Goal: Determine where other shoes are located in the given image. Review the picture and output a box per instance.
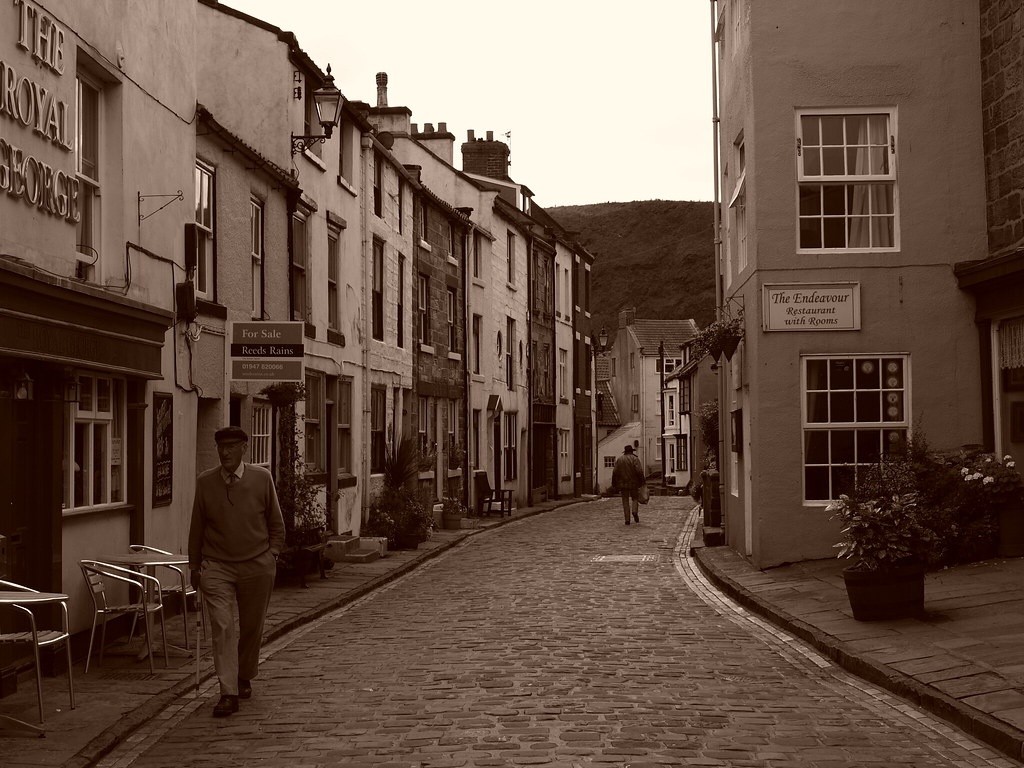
[632,512,639,523]
[625,521,630,525]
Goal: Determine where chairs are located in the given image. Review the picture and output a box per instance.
[78,559,169,675]
[476,471,515,518]
[126,544,207,651]
[0,580,75,723]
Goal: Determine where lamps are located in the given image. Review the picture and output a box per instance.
[590,326,608,356]
[291,63,345,158]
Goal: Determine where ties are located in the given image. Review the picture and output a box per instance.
[229,473,236,482]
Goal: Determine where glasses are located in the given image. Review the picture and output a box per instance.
[225,483,234,506]
[214,442,246,450]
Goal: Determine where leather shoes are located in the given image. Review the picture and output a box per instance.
[238,677,252,698]
[213,695,239,715]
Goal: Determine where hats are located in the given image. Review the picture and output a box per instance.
[622,445,636,453]
[215,426,248,443]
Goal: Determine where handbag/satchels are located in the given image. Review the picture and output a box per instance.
[638,483,650,504]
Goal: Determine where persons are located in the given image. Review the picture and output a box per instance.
[612,445,647,525]
[188,427,285,719]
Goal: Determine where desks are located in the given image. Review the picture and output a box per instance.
[95,556,193,661]
[0,592,70,738]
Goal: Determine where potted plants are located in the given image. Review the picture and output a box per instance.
[701,326,723,362]
[716,321,744,362]
[833,437,962,621]
